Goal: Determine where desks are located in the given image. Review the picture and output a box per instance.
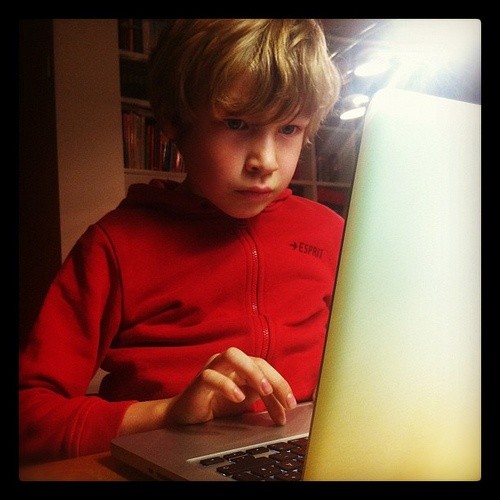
[19,450,154,481]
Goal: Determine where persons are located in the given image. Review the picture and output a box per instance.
[18,18,345,457]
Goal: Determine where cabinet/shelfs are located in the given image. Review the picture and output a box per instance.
[17,18,364,354]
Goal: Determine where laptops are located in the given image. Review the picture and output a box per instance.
[112,84,482,480]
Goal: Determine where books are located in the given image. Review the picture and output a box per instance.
[120,106,188,174]
[119,17,177,52]
[318,135,349,184]
